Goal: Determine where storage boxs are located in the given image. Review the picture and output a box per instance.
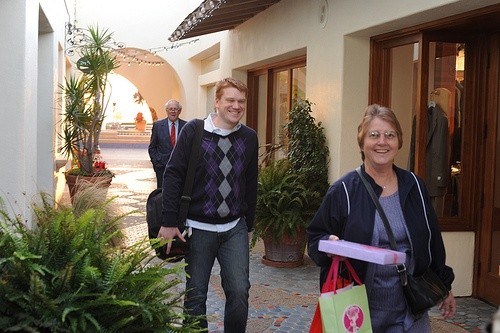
[318,240,407,265]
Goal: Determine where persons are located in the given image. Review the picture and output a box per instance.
[306,104,456,333]
[157,77,259,333]
[148,100,188,188]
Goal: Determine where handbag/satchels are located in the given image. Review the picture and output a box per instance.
[307,255,374,333]
[144,187,192,264]
[399,267,450,315]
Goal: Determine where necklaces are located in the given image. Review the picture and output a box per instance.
[383,186,387,189]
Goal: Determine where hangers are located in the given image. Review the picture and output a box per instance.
[410,91,449,120]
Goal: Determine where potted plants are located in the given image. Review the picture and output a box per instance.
[52,22,121,214]
[249,98,329,268]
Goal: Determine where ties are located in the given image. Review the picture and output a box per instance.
[170,122,176,147]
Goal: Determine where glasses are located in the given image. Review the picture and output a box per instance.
[367,129,398,139]
[169,108,179,113]
